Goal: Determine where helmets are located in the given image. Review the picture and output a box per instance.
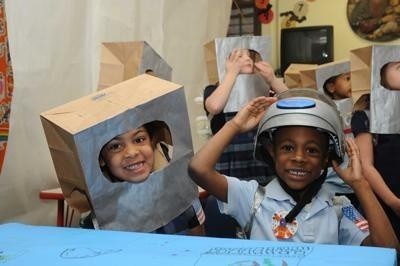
[253,88,346,167]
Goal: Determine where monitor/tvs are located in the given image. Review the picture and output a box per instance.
[281,25,334,78]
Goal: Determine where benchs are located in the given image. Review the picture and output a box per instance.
[39,186,66,227]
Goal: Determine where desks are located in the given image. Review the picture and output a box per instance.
[0,223,398,266]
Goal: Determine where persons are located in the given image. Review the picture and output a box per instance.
[67,46,400,247]
